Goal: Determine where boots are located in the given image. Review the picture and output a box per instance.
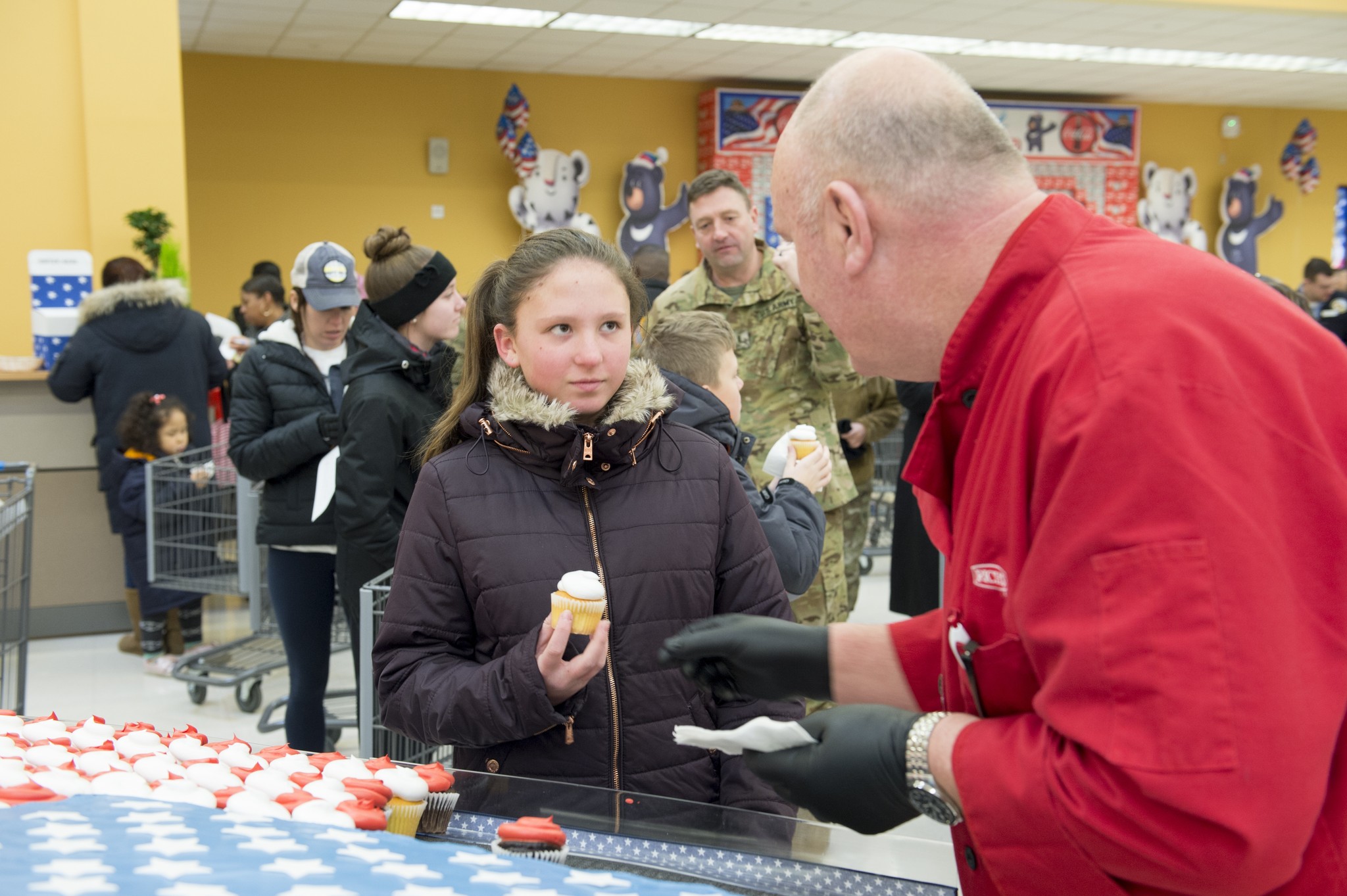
[117,587,186,656]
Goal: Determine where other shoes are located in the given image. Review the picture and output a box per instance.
[142,656,174,675]
[185,645,218,658]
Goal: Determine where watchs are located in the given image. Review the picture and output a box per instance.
[903,709,966,826]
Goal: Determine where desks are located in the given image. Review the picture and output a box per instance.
[0,706,959,895]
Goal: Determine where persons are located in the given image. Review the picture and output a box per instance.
[42,255,231,665]
[1292,257,1334,329]
[819,359,911,632]
[630,167,864,863]
[223,273,298,415]
[638,304,837,611]
[111,388,220,680]
[883,368,947,628]
[1315,249,1347,348]
[371,224,821,879]
[223,258,288,341]
[330,225,476,741]
[222,238,371,763]
[653,42,1347,896]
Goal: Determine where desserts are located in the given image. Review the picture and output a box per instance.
[492,814,569,864]
[0,706,459,838]
[550,570,607,635]
[789,424,819,461]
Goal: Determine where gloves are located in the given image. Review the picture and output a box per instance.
[659,614,832,703]
[718,703,929,837]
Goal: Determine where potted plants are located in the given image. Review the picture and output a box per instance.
[124,205,171,284]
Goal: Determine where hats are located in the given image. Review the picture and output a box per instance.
[292,242,363,310]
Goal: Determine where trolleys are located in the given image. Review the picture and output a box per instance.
[145,441,354,713]
[854,407,912,572]
